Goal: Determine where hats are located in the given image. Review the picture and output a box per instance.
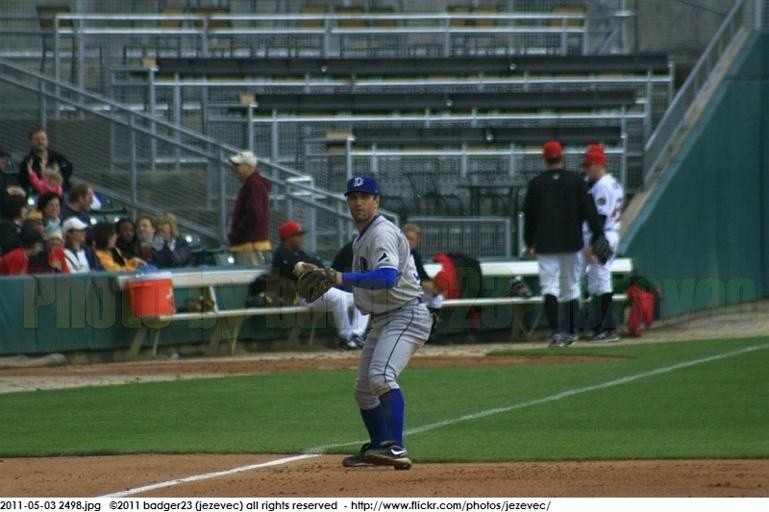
[542,140,563,159]
[279,220,310,239]
[63,215,89,234]
[344,175,379,198]
[229,150,258,168]
[580,144,608,166]
[47,224,63,241]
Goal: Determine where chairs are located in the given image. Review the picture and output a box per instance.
[0,149,239,267]
[353,169,543,218]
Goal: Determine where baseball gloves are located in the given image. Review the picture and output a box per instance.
[295,266,337,304]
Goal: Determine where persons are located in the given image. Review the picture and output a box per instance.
[228,151,272,352]
[402,223,440,343]
[290,173,433,469]
[0,125,192,276]
[522,140,624,349]
[273,220,371,350]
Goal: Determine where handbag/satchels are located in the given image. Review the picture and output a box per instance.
[245,271,303,309]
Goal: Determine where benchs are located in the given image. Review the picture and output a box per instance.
[113,250,636,358]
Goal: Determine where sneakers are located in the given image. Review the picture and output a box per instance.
[547,334,564,348]
[342,442,387,468]
[563,334,578,348]
[337,337,358,352]
[588,330,620,344]
[363,444,413,471]
[352,335,374,350]
